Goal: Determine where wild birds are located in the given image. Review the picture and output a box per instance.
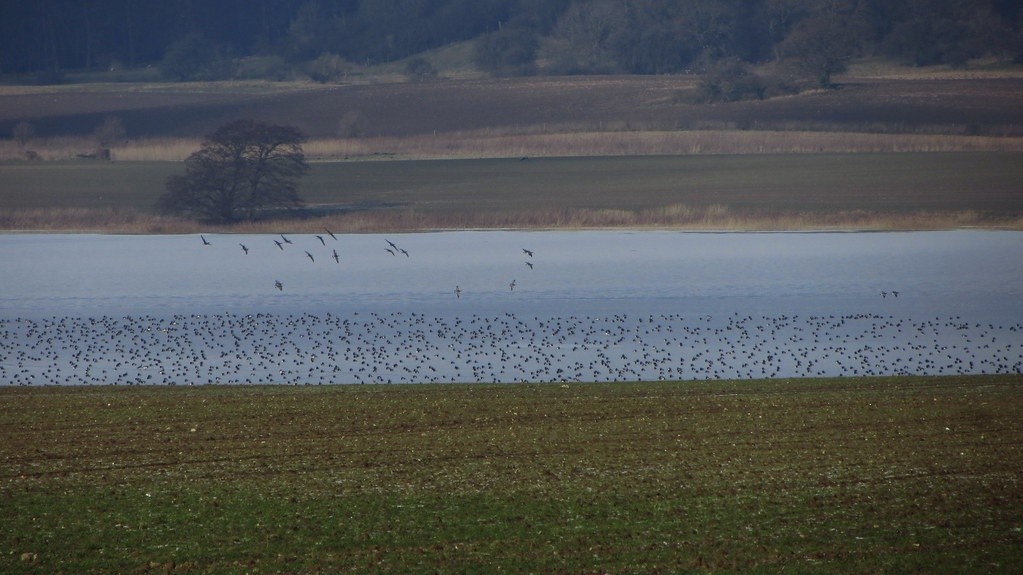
[399,248,409,257]
[892,290,899,297]
[315,235,325,246]
[332,249,341,263]
[240,243,249,255]
[384,248,395,257]
[201,235,214,245]
[304,250,314,263]
[454,285,464,299]
[880,291,887,298]
[509,280,516,291]
[522,248,535,257]
[0,312,1023,386]
[273,240,283,250]
[384,239,399,252]
[325,228,338,240]
[280,234,297,244]
[525,262,533,269]
[274,279,283,292]
